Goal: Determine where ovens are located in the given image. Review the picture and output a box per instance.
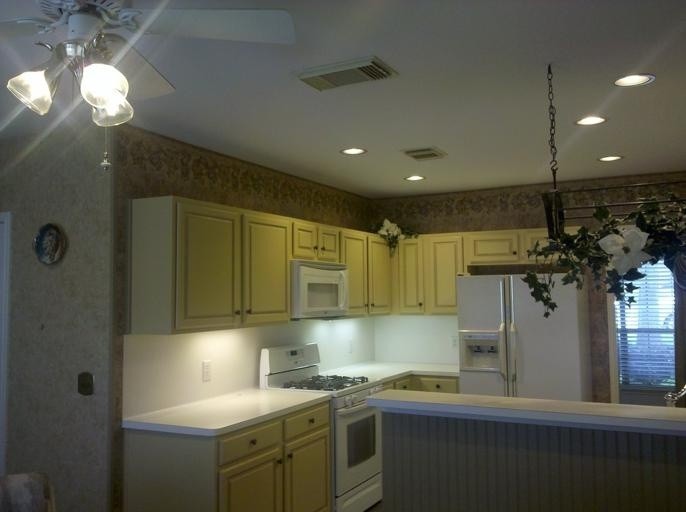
[334,399,384,495]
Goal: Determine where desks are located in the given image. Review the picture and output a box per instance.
[364,389,684,511]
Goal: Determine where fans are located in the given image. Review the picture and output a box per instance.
[0,0,299,105]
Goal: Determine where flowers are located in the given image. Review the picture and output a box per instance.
[597,225,655,279]
[359,209,424,258]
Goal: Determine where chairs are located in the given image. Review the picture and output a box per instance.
[0,466,58,511]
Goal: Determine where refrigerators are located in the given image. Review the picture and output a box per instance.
[454,273,595,403]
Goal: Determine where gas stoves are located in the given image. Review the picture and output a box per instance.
[265,366,383,410]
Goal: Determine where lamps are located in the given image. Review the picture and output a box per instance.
[5,13,135,174]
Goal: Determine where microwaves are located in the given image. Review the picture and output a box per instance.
[290,259,349,321]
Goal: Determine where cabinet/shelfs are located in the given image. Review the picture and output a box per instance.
[289,216,348,265]
[394,232,425,316]
[463,224,583,276]
[339,225,394,318]
[414,376,460,391]
[122,399,332,511]
[383,374,414,390]
[425,230,463,316]
[124,194,291,334]
